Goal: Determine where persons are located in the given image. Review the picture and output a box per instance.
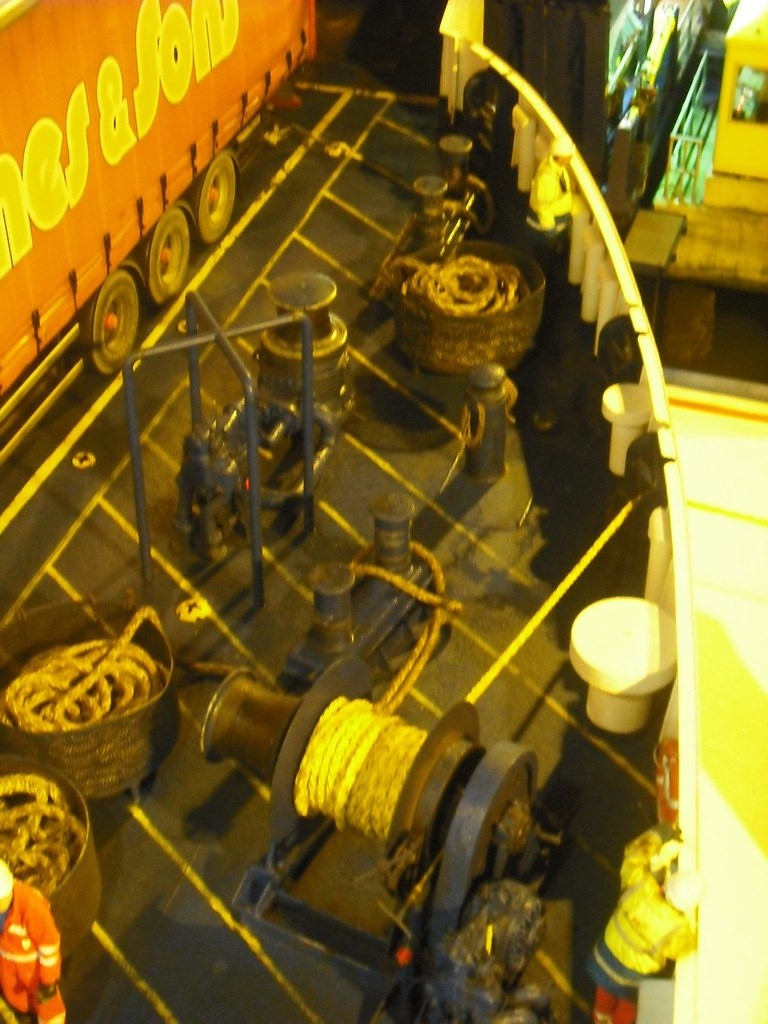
[0,858,66,1024]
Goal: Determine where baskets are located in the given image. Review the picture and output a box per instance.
[383,242,546,376]
[0,605,171,798]
